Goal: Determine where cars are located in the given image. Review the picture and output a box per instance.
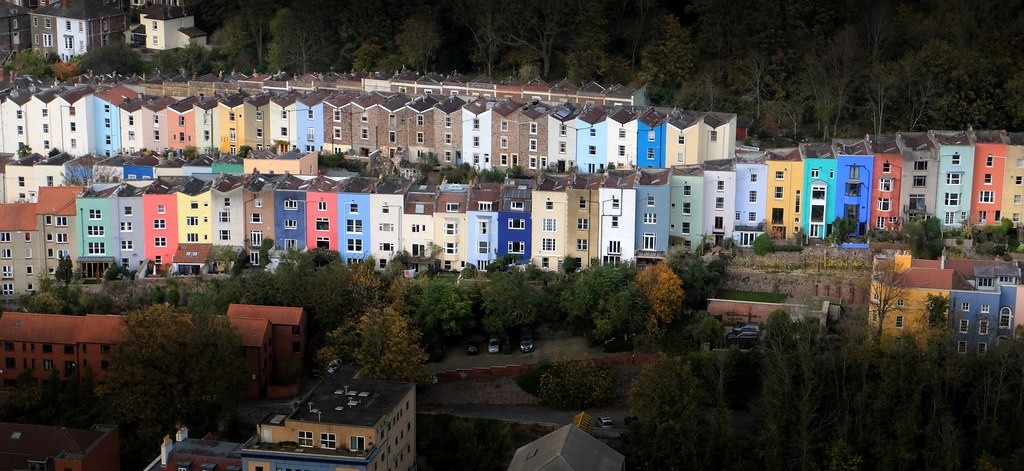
[519,335,534,353]
[327,358,343,373]
[726,330,758,343]
[464,341,480,356]
[596,416,615,428]
[624,415,641,428]
[733,324,760,336]
[488,338,501,354]
